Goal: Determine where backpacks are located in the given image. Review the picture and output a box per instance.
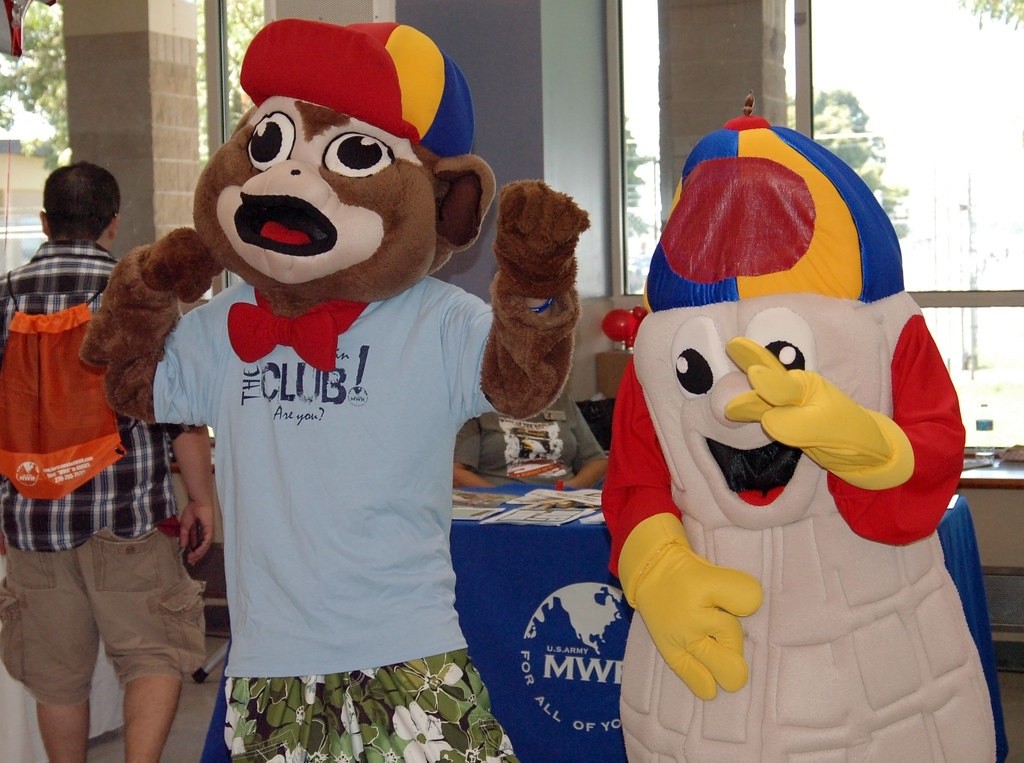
[0,271,126,500]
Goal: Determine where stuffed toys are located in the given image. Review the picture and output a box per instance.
[600,90,996,762]
[77,17,590,763]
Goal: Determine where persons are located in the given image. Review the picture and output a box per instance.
[0,163,216,763]
[453,392,608,491]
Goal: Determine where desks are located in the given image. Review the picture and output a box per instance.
[447,479,1013,763]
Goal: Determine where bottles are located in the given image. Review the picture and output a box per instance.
[975,403,994,467]
